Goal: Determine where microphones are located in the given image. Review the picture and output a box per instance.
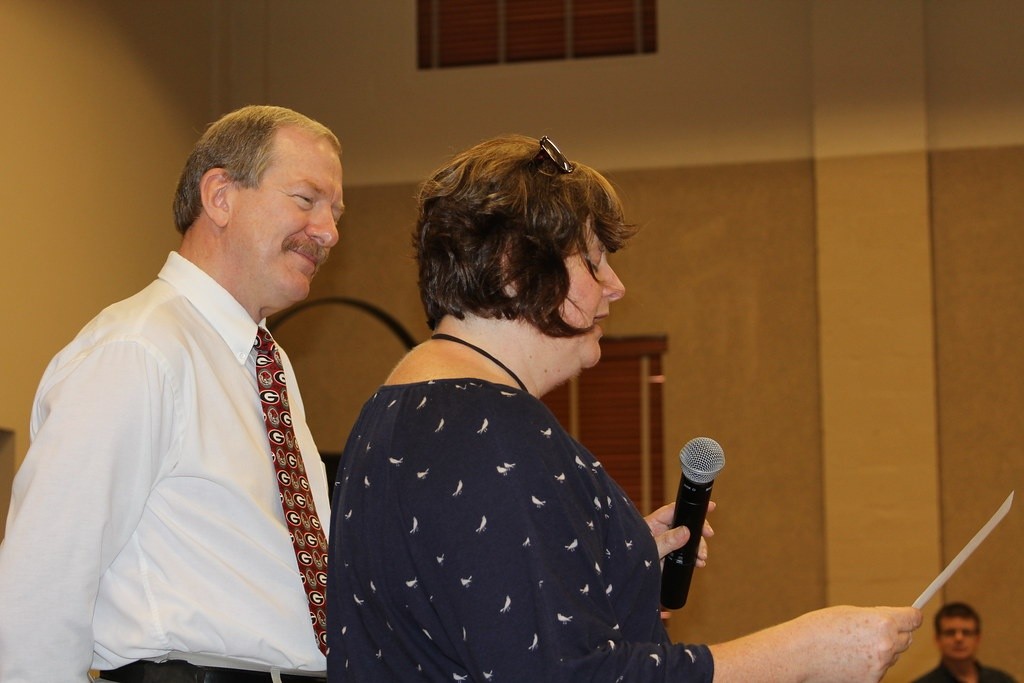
[660,436,726,610]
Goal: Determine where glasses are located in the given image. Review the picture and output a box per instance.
[529,136,575,174]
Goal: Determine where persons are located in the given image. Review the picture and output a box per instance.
[326,133,922,683]
[917,602,1019,682]
[0,107,345,683]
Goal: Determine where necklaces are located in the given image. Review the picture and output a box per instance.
[432,333,528,394]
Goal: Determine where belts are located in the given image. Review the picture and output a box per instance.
[98,659,328,683]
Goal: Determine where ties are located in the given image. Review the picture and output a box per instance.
[253,326,329,656]
